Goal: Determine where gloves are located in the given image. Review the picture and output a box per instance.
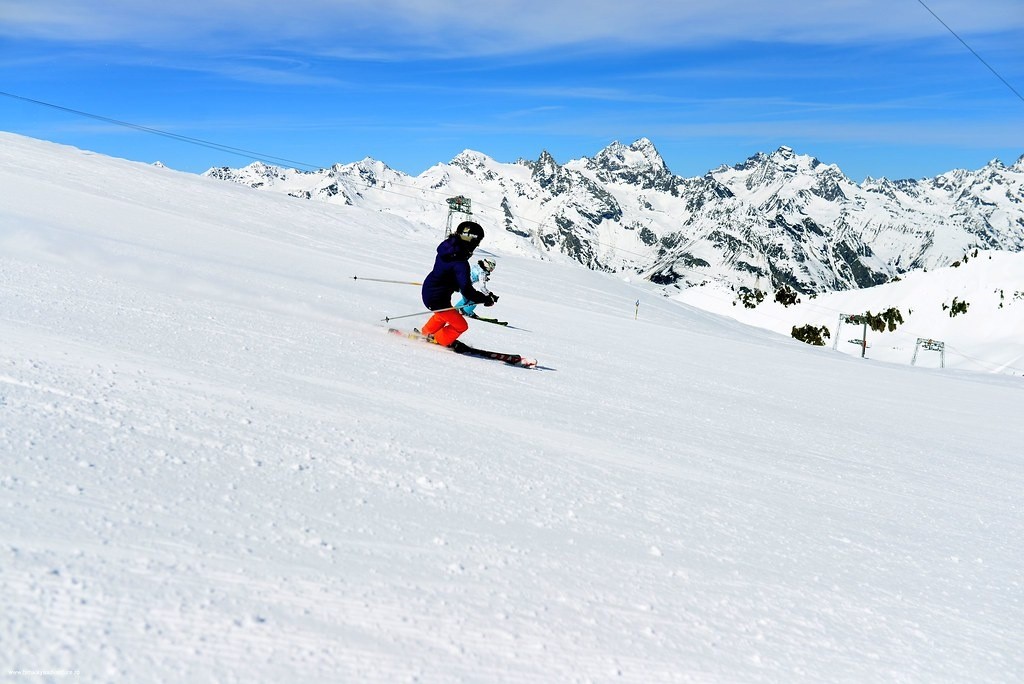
[484,296,494,306]
[489,292,498,302]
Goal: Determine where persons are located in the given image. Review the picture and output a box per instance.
[422,221,494,347]
[454,258,499,317]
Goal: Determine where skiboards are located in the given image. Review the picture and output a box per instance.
[389,329,558,371]
[455,311,530,331]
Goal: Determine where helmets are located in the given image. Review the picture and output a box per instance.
[484,258,496,271]
[457,221,484,243]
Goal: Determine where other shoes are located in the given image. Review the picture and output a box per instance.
[428,334,470,353]
[459,308,480,319]
